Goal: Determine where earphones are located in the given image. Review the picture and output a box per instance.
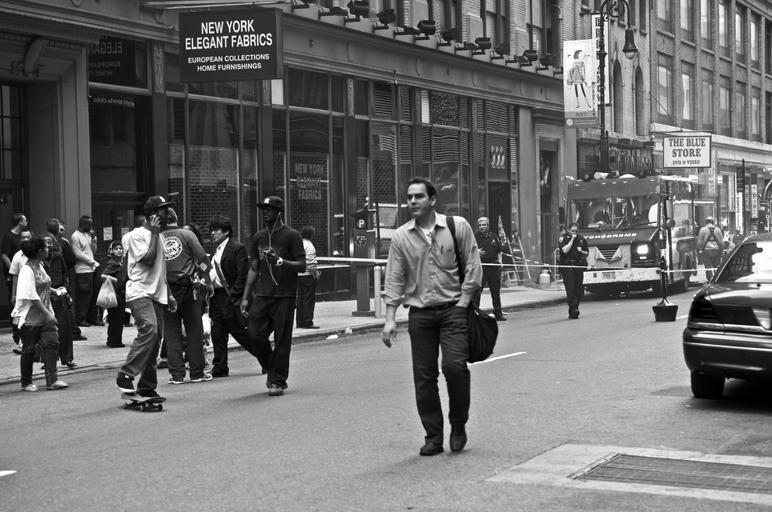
[278,211,282,216]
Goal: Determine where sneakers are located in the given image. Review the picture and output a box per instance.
[137,390,166,403]
[22,383,38,392]
[114,372,136,393]
[168,367,228,384]
[268,384,283,396]
[47,380,69,390]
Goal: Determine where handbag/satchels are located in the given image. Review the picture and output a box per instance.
[96,275,118,308]
[468,300,498,363]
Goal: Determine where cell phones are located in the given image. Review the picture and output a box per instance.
[264,250,270,255]
[149,214,157,220]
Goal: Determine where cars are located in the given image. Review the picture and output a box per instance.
[351,201,417,279]
[683,233,771,402]
[439,202,476,235]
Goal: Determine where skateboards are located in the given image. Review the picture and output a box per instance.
[121,393,168,411]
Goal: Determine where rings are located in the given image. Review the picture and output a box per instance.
[380,333,384,336]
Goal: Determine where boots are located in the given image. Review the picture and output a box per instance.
[492,309,506,321]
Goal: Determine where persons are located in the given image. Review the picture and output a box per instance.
[296,226,321,329]
[471,217,507,321]
[733,229,744,246]
[70,215,105,326]
[240,197,305,396]
[558,222,589,319]
[569,50,592,109]
[116,196,214,400]
[99,240,128,347]
[206,214,266,376]
[2,213,86,393]
[674,219,698,276]
[379,178,484,455]
[696,215,725,283]
[722,241,732,260]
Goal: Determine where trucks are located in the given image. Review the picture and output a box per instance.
[561,172,717,300]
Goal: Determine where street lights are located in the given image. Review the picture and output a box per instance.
[598,0,639,173]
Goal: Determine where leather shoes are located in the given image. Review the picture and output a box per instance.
[450,421,467,451]
[420,442,443,455]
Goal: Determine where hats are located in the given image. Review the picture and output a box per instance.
[144,195,175,216]
[256,195,284,209]
